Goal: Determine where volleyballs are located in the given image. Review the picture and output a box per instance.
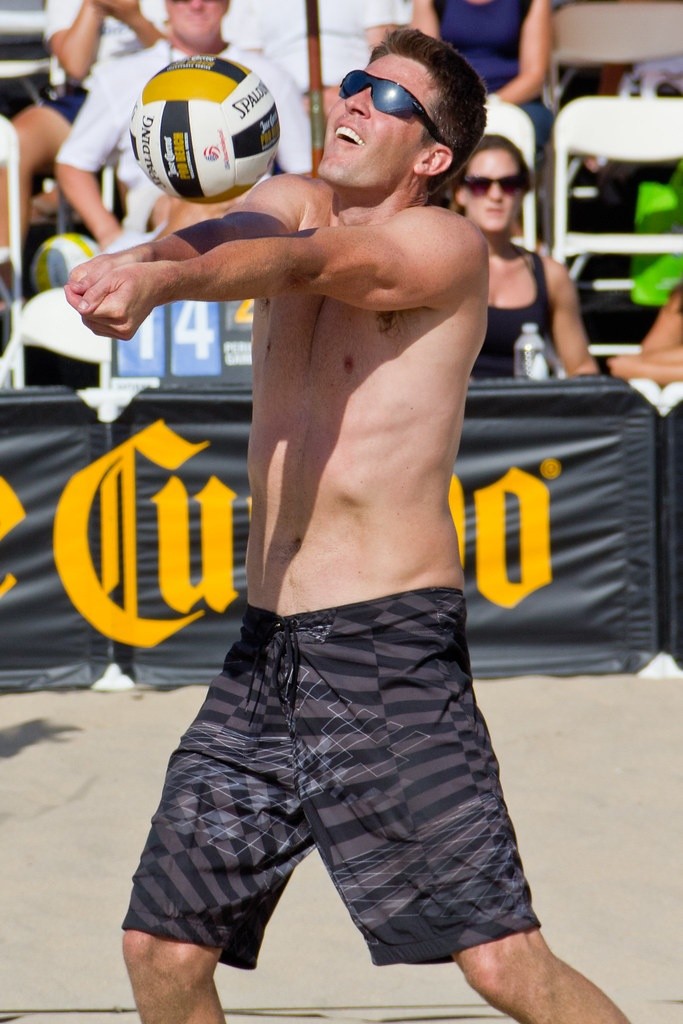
[129,56,277,205]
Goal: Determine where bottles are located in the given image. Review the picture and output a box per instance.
[512,322,547,382]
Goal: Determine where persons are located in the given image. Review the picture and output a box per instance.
[64,29,632,1024]
[0,0,683,384]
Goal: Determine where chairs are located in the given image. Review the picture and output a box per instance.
[481,96,538,254]
[0,0,116,389]
[547,0,683,114]
[551,93,683,375]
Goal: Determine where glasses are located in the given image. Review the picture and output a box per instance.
[340,70,448,148]
[463,174,526,196]
